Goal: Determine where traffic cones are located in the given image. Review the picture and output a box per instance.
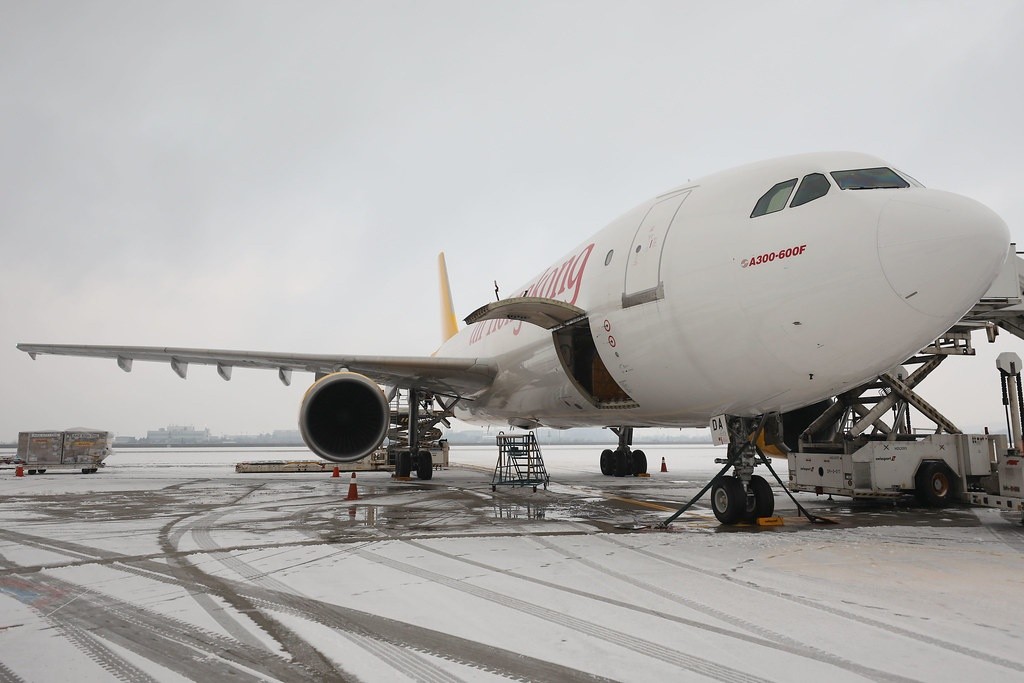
[342,472,363,500]
[13,460,26,477]
[330,466,342,478]
[660,457,670,472]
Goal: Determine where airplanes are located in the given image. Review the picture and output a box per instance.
[15,151,1011,525]
